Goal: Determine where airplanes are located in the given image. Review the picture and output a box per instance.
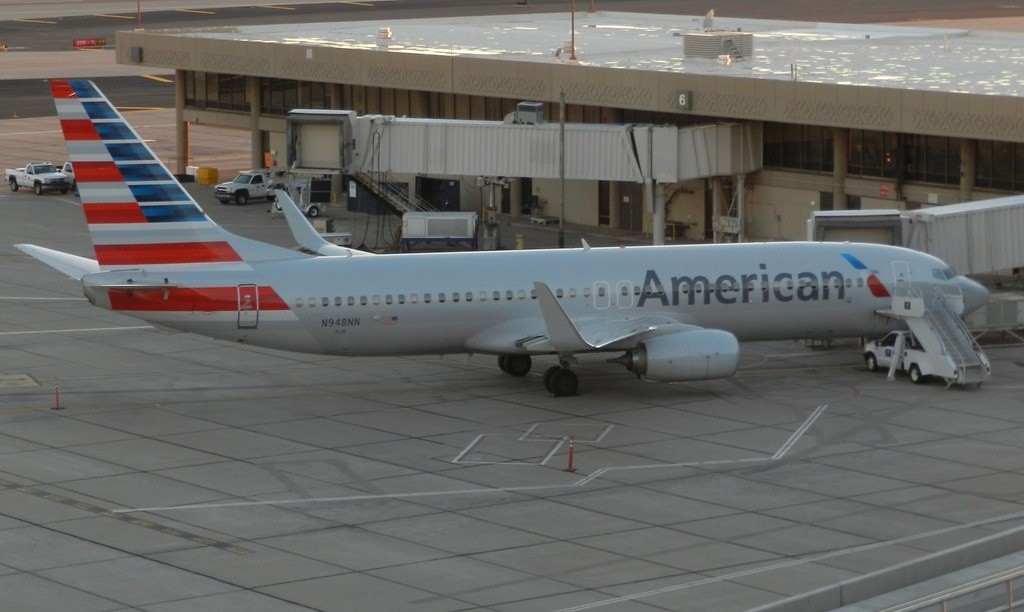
[9,78,994,397]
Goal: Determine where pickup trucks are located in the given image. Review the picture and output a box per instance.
[4,160,69,196]
[212,171,289,205]
[56,162,79,197]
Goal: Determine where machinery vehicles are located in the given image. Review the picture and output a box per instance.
[863,331,992,388]
